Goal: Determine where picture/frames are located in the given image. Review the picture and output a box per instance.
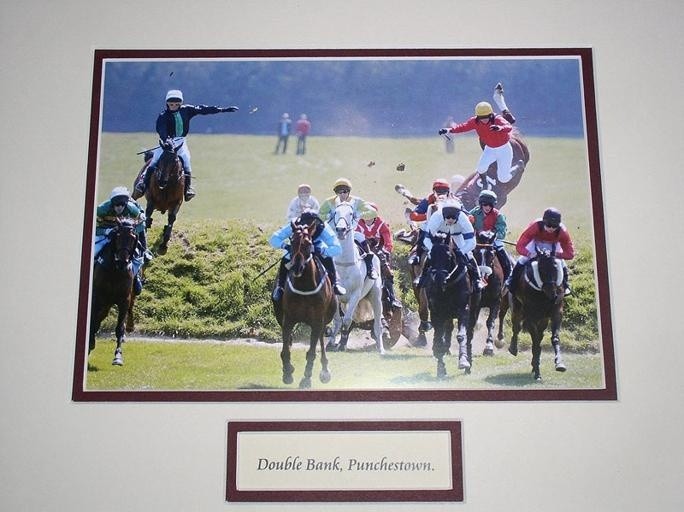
[225,421,464,502]
[72,48,619,401]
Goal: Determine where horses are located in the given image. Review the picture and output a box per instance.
[508,241,567,381]
[395,227,517,378]
[324,196,386,355]
[131,139,186,256]
[85,218,144,367]
[271,218,332,390]
[393,82,530,211]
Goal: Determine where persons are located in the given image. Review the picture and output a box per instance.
[437,102,526,191]
[271,112,292,155]
[95,186,150,294]
[294,113,311,155]
[134,89,239,202]
[265,174,576,335]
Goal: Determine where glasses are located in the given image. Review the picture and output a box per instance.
[338,189,349,193]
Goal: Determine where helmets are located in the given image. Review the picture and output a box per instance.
[474,102,493,116]
[543,207,561,225]
[432,180,497,217]
[333,178,350,190]
[110,186,129,201]
[165,90,183,103]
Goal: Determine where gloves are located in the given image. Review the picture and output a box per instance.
[488,124,501,132]
[222,106,239,112]
[438,127,450,135]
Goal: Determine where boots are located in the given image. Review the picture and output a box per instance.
[508,261,523,292]
[510,160,525,177]
[134,166,155,191]
[363,254,374,278]
[479,173,487,190]
[383,279,394,301]
[185,171,195,201]
[470,259,481,290]
